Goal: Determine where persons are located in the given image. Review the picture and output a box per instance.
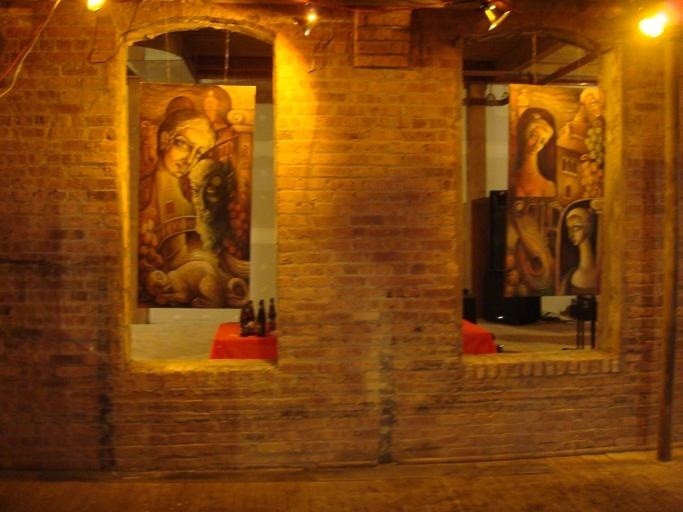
[566,208,598,289]
[139,85,249,308]
[512,112,556,197]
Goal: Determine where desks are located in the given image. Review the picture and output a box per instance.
[209,320,497,359]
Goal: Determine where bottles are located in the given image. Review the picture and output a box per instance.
[238,298,277,339]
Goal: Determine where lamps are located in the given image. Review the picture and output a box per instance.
[480,0,510,31]
[293,1,318,38]
